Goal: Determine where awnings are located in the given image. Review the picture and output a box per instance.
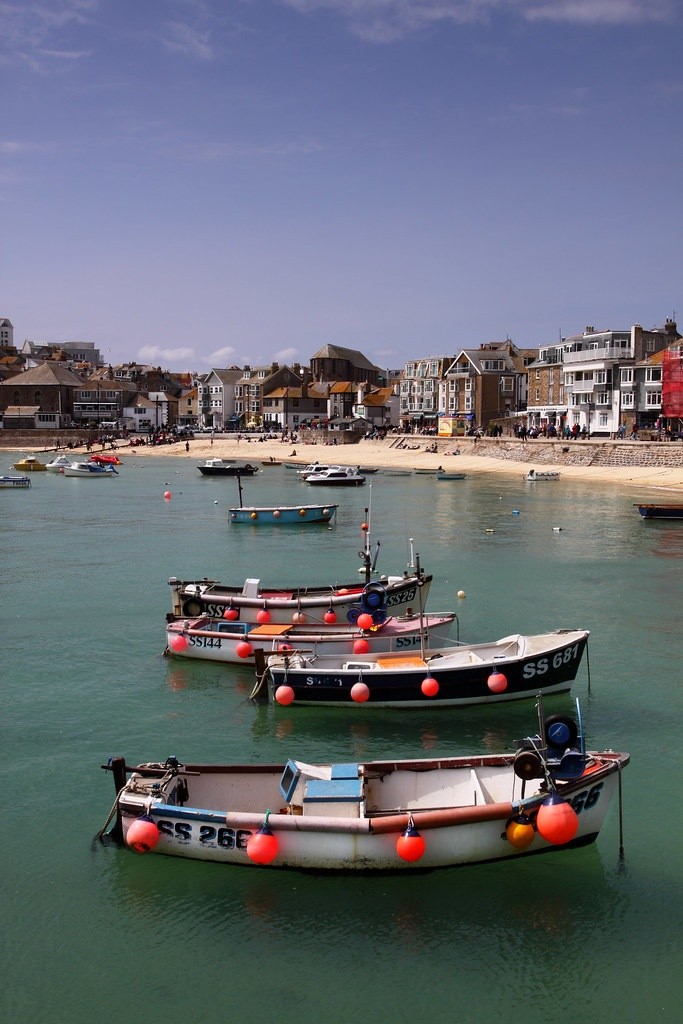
[526,412,540,417]
[409,413,438,420]
[557,411,566,416]
[545,412,556,417]
[465,414,473,421]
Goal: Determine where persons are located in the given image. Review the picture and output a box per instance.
[290,450,296,456]
[617,422,639,440]
[640,421,683,441]
[237,422,328,444]
[56,433,118,452]
[123,421,194,447]
[466,422,586,440]
[103,423,113,430]
[211,436,213,445]
[364,424,438,440]
[398,444,460,455]
[185,441,189,452]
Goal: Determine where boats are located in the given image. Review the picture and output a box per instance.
[14,457,46,472]
[384,468,412,477]
[633,501,682,521]
[285,462,380,485]
[526,468,560,481]
[414,468,443,475]
[163,607,457,664]
[261,456,282,466]
[167,508,433,622]
[0,476,32,488]
[436,471,466,481]
[228,468,339,526]
[63,460,116,478]
[196,457,259,476]
[248,556,592,707]
[90,450,124,465]
[46,452,71,472]
[100,691,631,875]
